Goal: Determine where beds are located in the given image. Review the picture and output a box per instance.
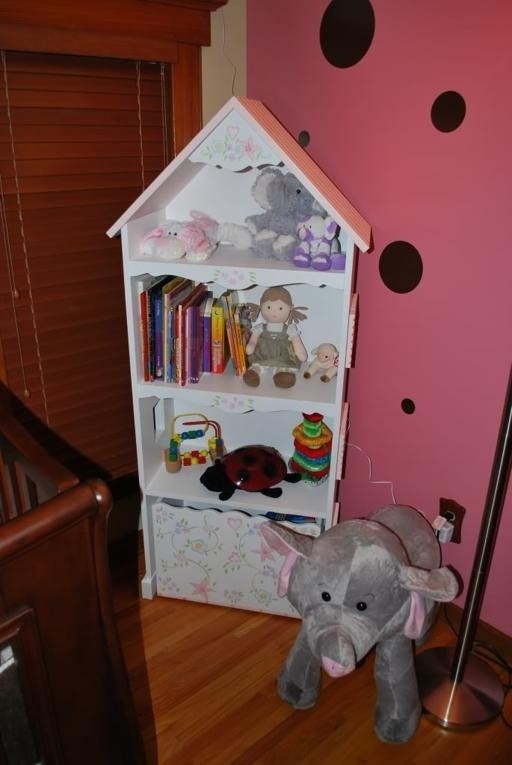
[1,387,121,762]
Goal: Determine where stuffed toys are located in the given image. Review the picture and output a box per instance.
[246,166,327,262]
[260,505,461,747]
[142,211,252,262]
[304,343,339,383]
[200,444,301,501]
[290,214,348,272]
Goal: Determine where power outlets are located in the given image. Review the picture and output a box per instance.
[431,497,467,546]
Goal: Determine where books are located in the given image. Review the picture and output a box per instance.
[135,272,252,388]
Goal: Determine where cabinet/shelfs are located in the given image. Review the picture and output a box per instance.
[1,0,228,587]
[106,92,372,622]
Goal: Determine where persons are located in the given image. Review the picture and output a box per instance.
[244,285,307,389]
